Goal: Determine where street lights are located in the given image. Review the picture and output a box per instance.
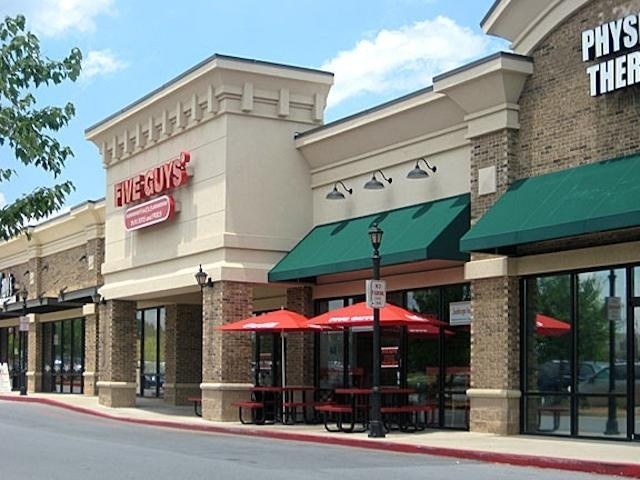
[20,285,28,394]
[366,221,388,437]
[91,286,101,397]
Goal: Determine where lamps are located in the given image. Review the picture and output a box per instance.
[194,264,213,289]
[326,157,437,200]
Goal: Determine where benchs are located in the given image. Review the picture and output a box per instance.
[536,406,570,432]
[232,403,435,438]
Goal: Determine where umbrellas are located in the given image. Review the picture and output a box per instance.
[535,313,573,338]
[404,325,454,336]
[308,301,450,326]
[214,306,348,423]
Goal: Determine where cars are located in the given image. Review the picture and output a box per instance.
[141,373,163,389]
[537,359,639,407]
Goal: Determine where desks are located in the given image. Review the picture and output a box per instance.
[249,385,316,424]
[336,387,417,433]
